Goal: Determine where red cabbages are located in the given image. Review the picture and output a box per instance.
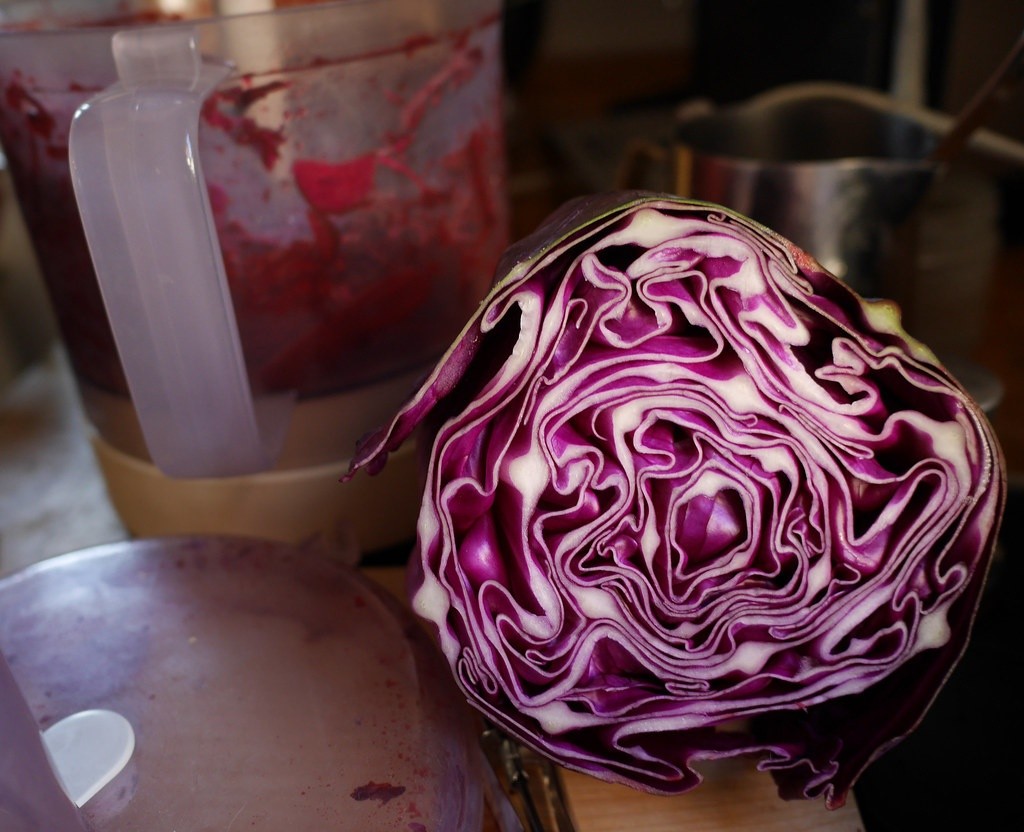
[348,196,1004,811]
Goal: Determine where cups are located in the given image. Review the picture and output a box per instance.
[636,82,944,333]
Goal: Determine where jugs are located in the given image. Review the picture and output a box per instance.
[0,1,506,556]
